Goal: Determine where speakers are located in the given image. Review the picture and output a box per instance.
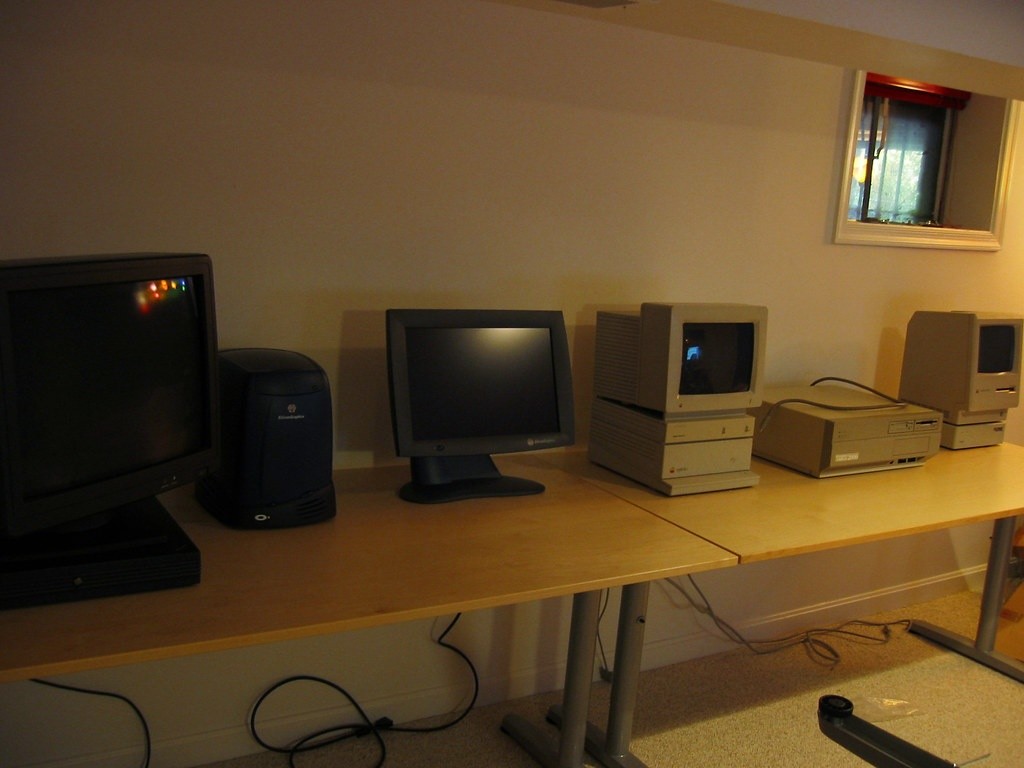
[197,348,338,531]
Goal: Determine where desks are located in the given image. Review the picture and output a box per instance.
[0,444,1024,768]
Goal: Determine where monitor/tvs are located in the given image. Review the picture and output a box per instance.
[597,302,768,414]
[899,309,1024,424]
[384,309,576,505]
[0,252,219,562]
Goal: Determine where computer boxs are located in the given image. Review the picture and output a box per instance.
[0,496,201,610]
